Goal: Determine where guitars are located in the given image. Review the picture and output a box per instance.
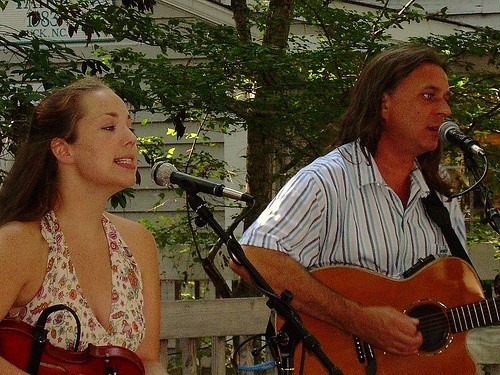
[0,318,145,375]
[274,256,500,375]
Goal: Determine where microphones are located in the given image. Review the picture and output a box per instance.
[151,161,254,203]
[438,121,485,158]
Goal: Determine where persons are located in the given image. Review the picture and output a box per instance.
[0,76,170,375]
[228,45,467,375]
